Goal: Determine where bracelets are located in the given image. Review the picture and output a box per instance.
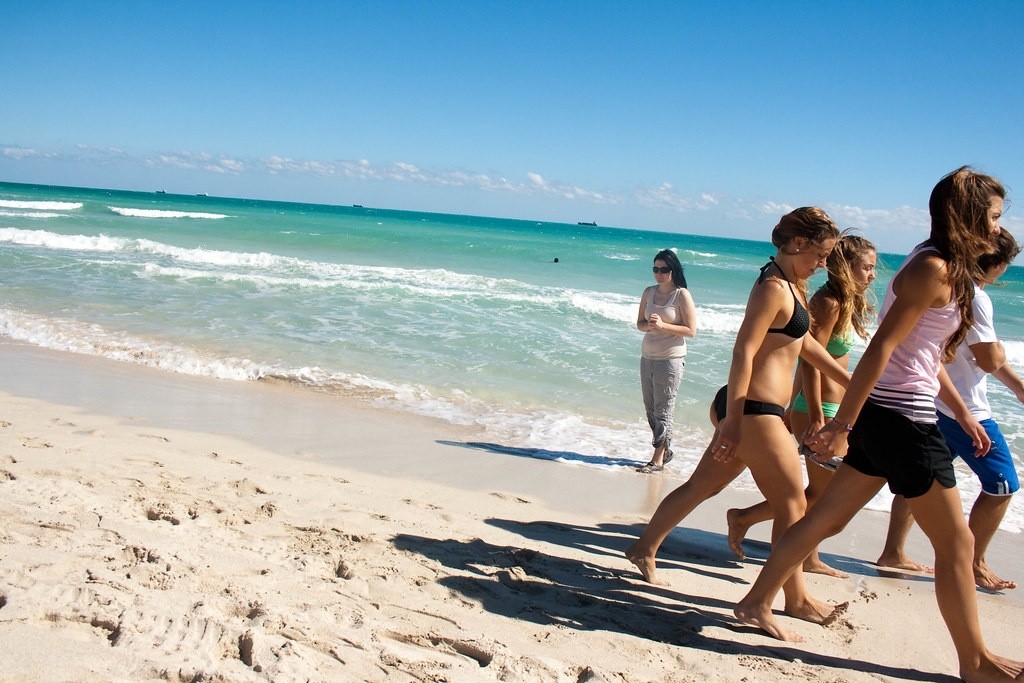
[833,418,852,431]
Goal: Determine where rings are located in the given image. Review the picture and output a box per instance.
[721,445,726,449]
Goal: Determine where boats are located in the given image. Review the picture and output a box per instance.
[578,221,598,226]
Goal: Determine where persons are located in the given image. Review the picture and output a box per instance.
[637,249,696,476]
[625,164,1024,683]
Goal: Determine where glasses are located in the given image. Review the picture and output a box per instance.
[653,266,672,273]
